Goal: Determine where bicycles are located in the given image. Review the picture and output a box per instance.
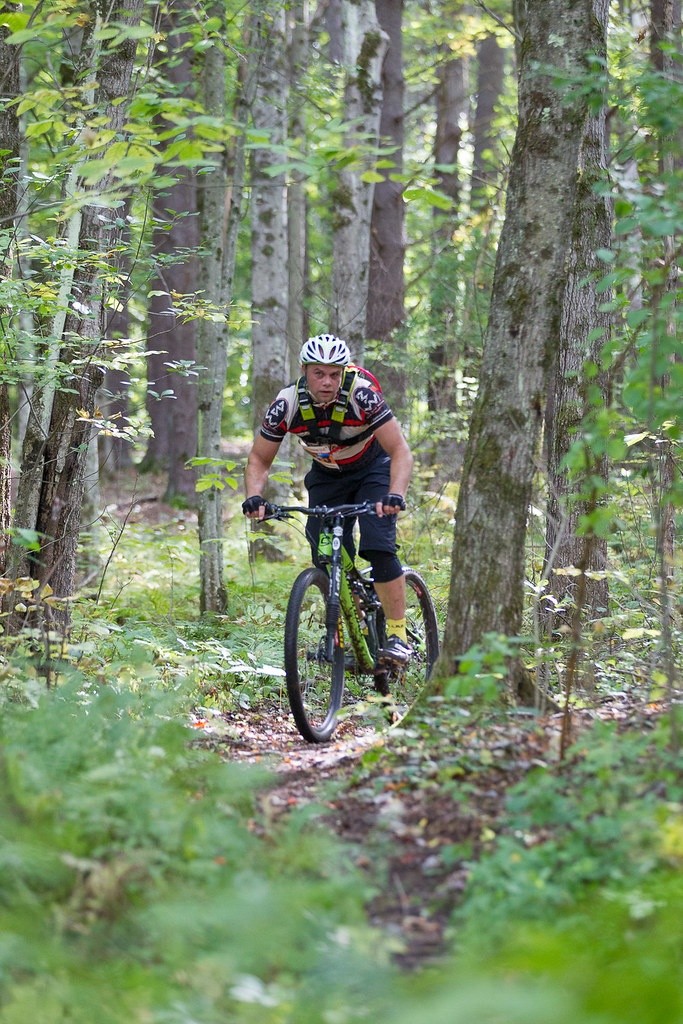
[248,498,441,746]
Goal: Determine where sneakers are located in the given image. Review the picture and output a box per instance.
[375,633,410,667]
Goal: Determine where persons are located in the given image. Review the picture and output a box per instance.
[242,334,414,667]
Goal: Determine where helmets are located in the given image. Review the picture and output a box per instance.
[300,333,351,367]
